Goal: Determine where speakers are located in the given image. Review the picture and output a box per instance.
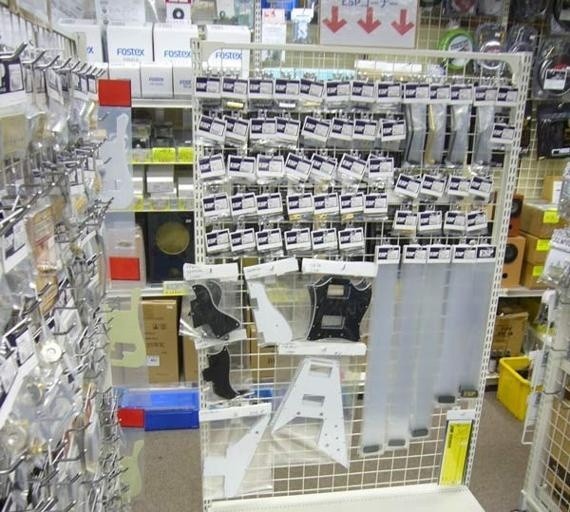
[148,211,195,283]
[500,236,525,293]
[507,195,524,237]
